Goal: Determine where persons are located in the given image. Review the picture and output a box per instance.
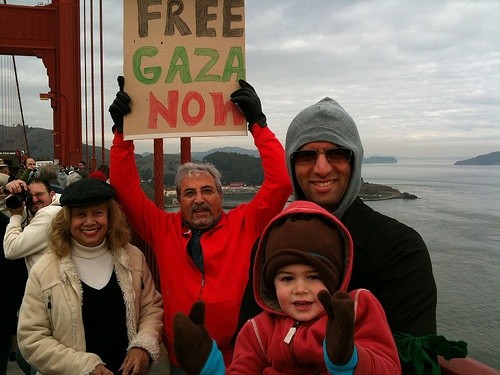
[109,76,293,375]
[0,150,112,375]
[17,177,165,375]
[234,98,441,375]
[229,199,402,375]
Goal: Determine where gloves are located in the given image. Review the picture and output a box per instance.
[318,289,355,366]
[173,301,214,375]
[230,79,267,131]
[109,76,131,134]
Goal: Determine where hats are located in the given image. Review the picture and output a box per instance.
[262,214,344,295]
[60,178,116,208]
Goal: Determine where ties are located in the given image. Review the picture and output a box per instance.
[187,229,208,273]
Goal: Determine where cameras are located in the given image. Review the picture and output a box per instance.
[5,186,29,209]
[33,166,39,171]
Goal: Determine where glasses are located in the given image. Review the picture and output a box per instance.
[290,147,352,165]
